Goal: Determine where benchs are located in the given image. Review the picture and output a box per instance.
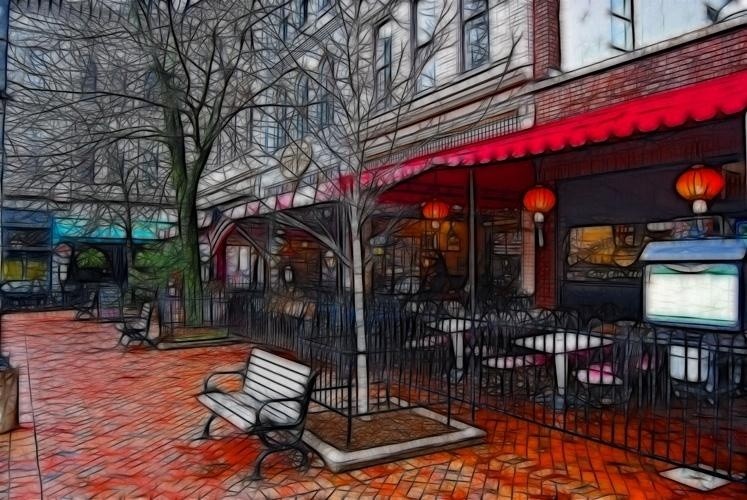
[194,346,322,480]
[114,301,154,349]
[74,290,97,321]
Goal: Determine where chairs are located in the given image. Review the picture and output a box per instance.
[403,301,660,410]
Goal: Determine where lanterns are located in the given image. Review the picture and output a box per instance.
[674,164,724,214]
[422,198,448,229]
[523,185,555,223]
